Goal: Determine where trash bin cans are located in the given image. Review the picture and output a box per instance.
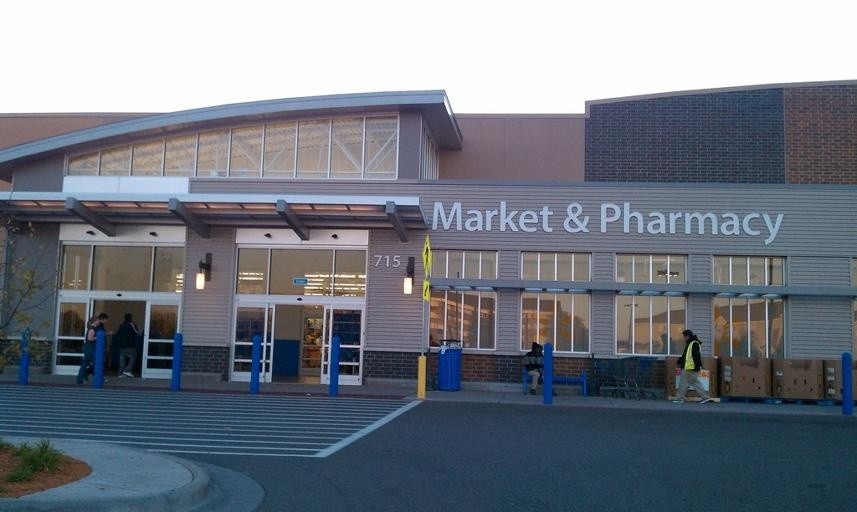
[439,339,460,390]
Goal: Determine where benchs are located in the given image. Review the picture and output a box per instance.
[521,366,587,397]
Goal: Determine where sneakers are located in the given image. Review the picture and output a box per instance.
[672,399,684,403]
[118,372,134,378]
[530,389,536,395]
[700,398,710,403]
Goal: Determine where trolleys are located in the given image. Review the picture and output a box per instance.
[610,356,659,401]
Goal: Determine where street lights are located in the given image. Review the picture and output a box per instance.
[624,303,638,354]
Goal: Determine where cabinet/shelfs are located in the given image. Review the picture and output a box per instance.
[234,318,271,365]
[302,314,360,368]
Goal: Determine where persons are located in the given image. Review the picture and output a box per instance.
[304,330,322,368]
[523,341,546,396]
[660,333,673,354]
[77,316,111,385]
[83,312,113,384]
[429,335,439,347]
[670,329,712,404]
[115,312,141,378]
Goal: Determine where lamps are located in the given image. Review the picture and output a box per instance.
[403,257,415,295]
[657,270,680,278]
[195,253,212,290]
